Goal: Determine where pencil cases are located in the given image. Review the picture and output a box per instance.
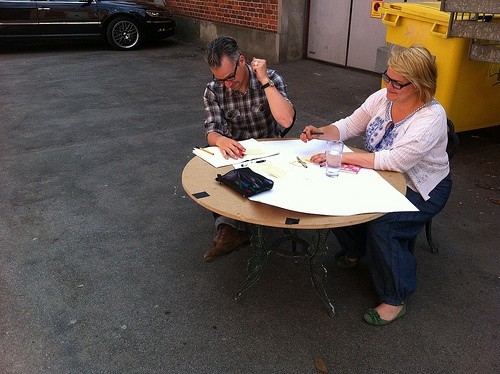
[214,167,274,199]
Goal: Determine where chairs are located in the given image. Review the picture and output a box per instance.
[411,118,455,255]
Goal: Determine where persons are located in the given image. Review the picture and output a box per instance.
[202,35,296,262]
[299,44,452,326]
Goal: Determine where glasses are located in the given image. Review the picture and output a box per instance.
[381,70,413,90]
[213,53,242,84]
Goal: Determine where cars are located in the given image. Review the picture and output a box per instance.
[0,0,179,50]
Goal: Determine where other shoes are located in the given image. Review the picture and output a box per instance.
[363,300,407,325]
[337,245,376,268]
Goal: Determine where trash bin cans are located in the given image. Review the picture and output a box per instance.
[376,2,500,133]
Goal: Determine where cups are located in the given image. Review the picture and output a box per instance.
[326,140,344,178]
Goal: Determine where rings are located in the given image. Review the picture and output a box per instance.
[254,62,258,66]
[324,156,327,161]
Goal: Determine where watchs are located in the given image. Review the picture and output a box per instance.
[261,80,275,89]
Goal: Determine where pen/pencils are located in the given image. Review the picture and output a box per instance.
[238,148,242,156]
[301,132,324,135]
[197,146,214,155]
[297,157,307,168]
[225,169,272,194]
[242,159,267,168]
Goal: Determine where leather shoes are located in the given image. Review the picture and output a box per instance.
[203,233,226,263]
[214,223,243,254]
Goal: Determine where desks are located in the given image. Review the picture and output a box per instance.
[182,138,407,316]
